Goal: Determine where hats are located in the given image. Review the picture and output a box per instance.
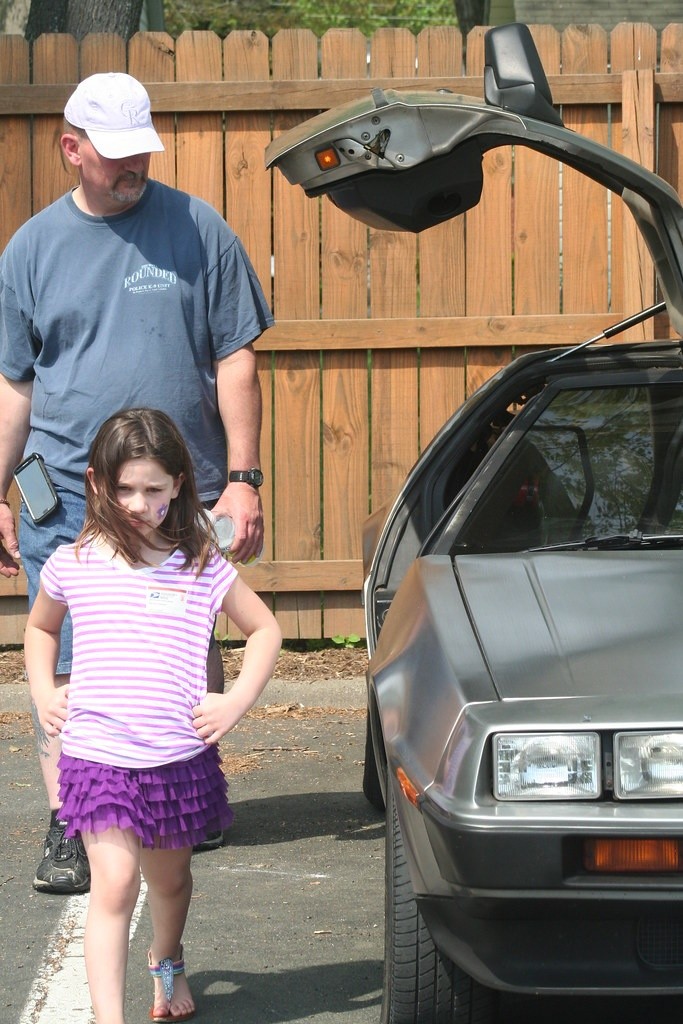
[62,72,165,160]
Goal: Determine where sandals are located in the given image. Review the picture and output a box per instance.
[147,942,196,1023]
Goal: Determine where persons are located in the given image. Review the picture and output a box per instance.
[2,69,274,892]
[21,407,283,1023]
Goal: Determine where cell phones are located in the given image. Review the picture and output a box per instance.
[12,453,61,524]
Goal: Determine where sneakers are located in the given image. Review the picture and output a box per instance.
[196,832,222,852]
[33,826,91,893]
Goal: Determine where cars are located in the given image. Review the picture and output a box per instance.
[268,23,683,1022]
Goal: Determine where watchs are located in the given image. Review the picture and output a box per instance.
[229,467,264,489]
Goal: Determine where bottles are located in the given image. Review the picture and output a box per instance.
[195,507,264,568]
